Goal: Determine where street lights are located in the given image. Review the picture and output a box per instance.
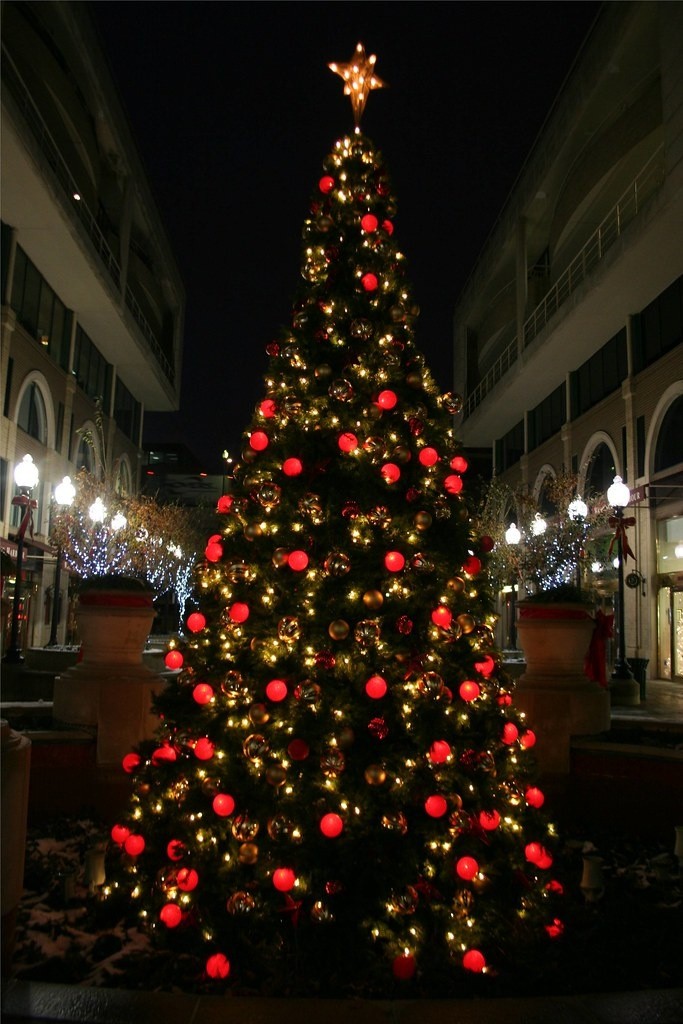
[43,475,77,650]
[497,522,525,661]
[566,493,590,593]
[1,454,39,665]
[605,474,644,708]
[87,495,108,573]
